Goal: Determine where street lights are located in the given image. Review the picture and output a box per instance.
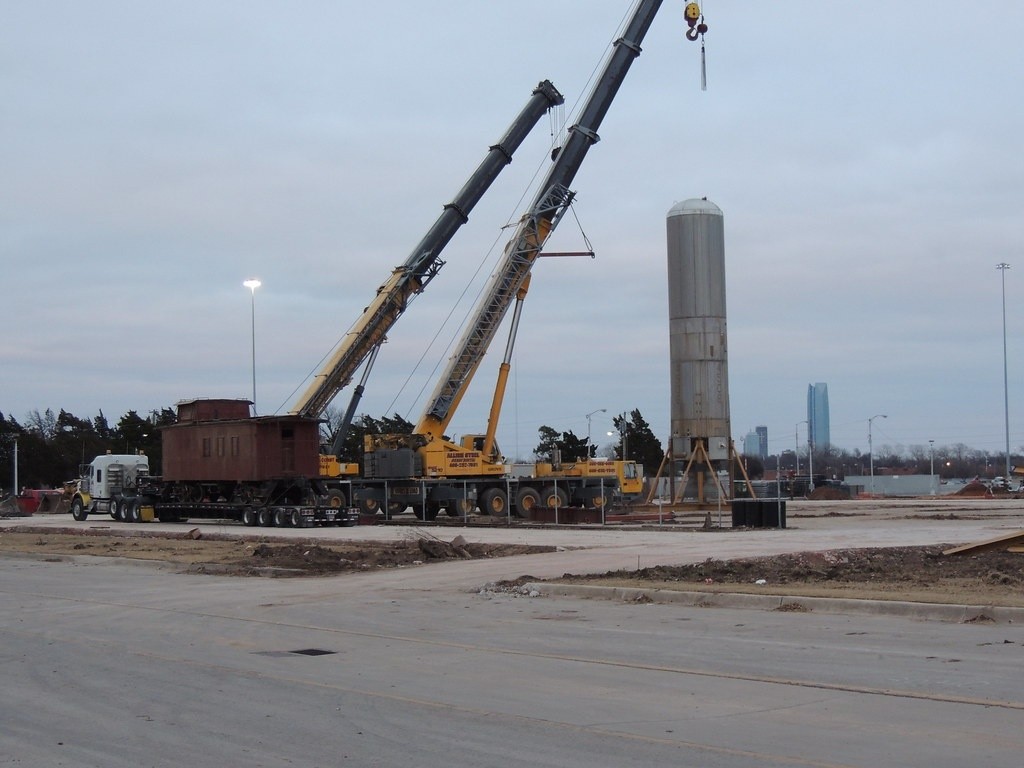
[9,433,20,496]
[996,262,1013,483]
[586,409,607,457]
[795,420,809,476]
[929,439,936,496]
[867,414,887,497]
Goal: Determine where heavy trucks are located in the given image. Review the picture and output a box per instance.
[70,450,360,528]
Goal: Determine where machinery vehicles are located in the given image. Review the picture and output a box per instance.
[34,464,90,514]
[506,448,646,515]
[352,0,707,521]
[287,80,565,520]
